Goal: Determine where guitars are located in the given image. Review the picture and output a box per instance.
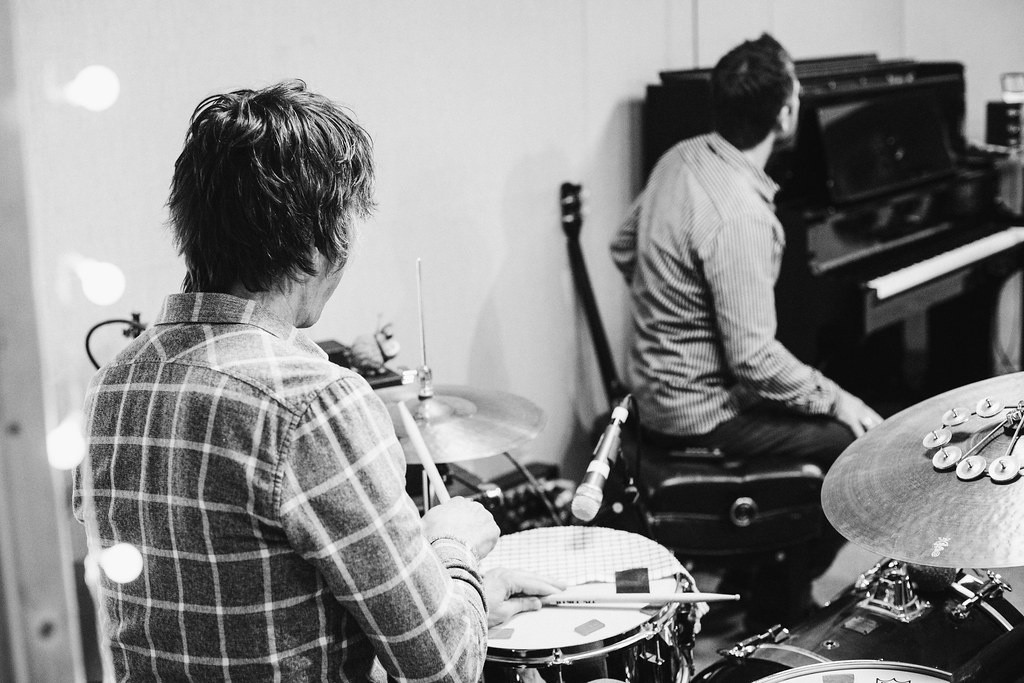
[558,178,648,490]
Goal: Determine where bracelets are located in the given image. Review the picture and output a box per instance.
[429,534,479,566]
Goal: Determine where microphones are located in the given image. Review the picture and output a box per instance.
[571,397,630,522]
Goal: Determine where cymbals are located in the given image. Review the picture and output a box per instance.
[821,370,1023,569]
[373,364,548,468]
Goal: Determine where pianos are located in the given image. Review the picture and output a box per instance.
[639,49,1024,364]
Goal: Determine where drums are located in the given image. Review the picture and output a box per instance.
[482,524,695,683]
[738,554,1023,675]
[748,657,961,683]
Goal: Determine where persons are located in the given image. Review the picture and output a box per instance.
[607,30,886,626]
[73,77,567,683]
